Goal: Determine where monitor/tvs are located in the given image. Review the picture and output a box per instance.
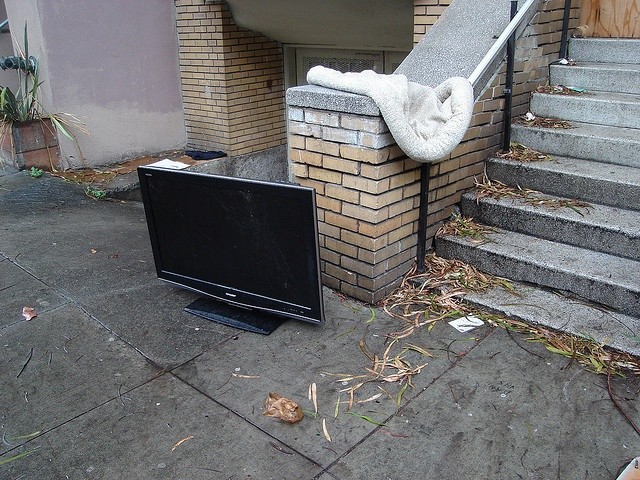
[137,165,327,337]
